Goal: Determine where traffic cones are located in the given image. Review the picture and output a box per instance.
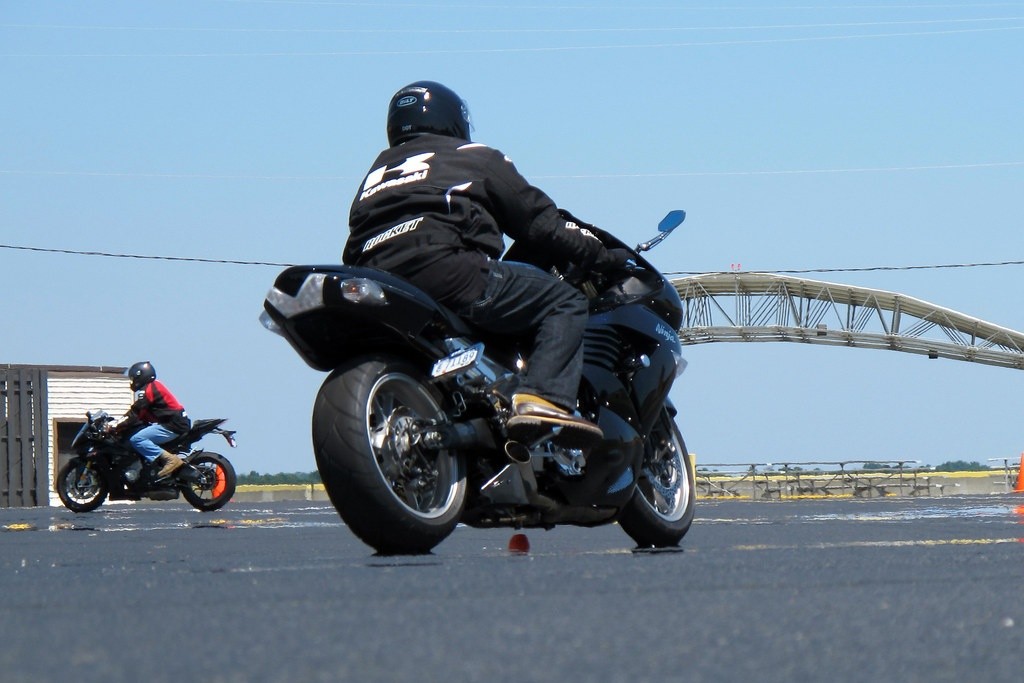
[1013,452,1024,494]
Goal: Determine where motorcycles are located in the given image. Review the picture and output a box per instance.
[260,210,698,556]
[56,409,239,512]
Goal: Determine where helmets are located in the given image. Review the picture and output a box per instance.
[127,360,157,393]
[386,80,472,147]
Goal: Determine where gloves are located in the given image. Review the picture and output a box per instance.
[103,420,118,434]
[592,245,638,283]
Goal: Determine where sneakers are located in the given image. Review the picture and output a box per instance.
[157,454,183,476]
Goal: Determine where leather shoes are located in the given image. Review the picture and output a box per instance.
[505,392,604,450]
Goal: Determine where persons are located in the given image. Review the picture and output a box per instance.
[340,82,636,451]
[106,361,192,479]
